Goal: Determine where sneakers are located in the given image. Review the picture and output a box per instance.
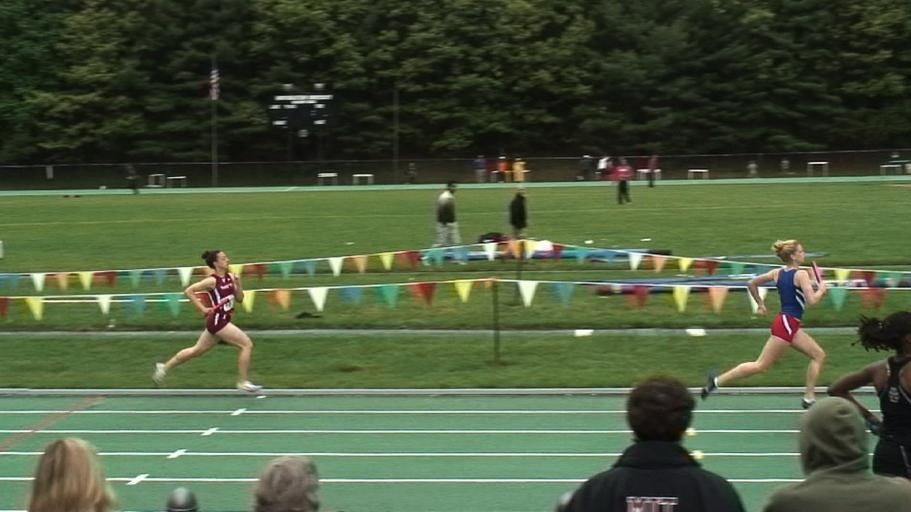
[153,361,166,388]
[701,373,716,400]
[236,380,262,393]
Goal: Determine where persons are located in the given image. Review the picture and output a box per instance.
[700,239,826,409]
[827,311,911,480]
[122,165,141,194]
[152,250,263,392]
[763,396,911,512]
[579,154,658,205]
[421,179,466,267]
[25,438,116,512]
[557,377,746,512]
[167,488,195,511]
[254,456,319,512]
[473,154,528,182]
[510,186,527,237]
